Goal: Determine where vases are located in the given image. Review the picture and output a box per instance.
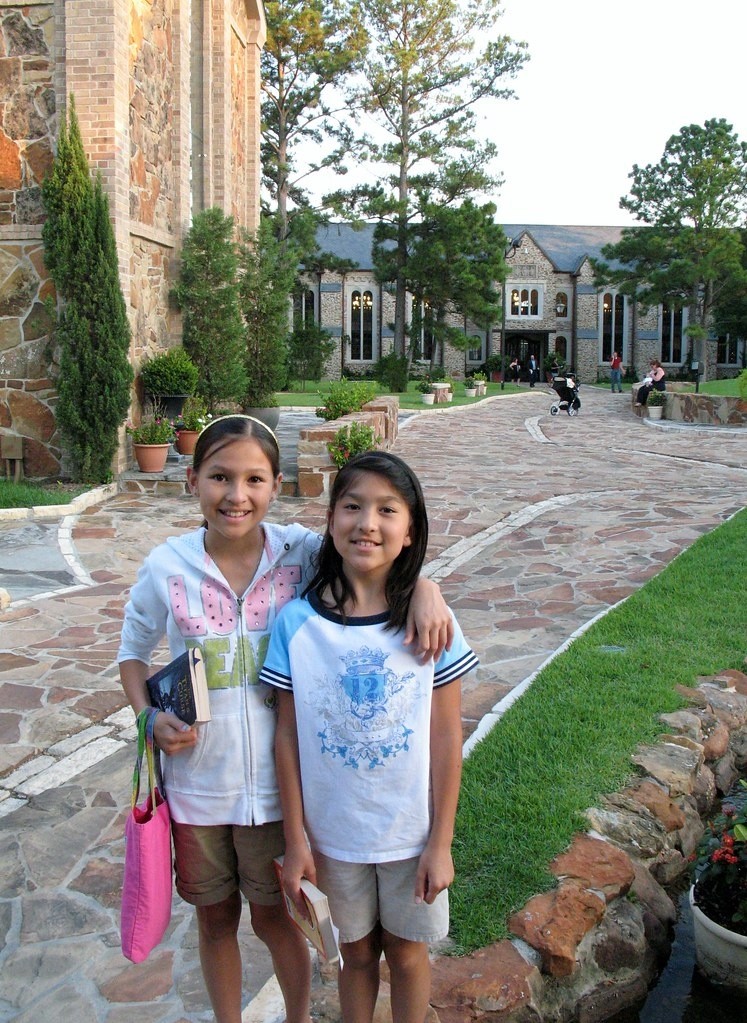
[177,431,199,455]
[689,878,747,992]
[132,443,171,472]
[647,407,663,420]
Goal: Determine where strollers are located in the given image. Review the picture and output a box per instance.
[551,372,581,416]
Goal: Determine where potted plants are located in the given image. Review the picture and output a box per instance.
[485,355,511,382]
[437,377,456,402]
[542,351,567,382]
[463,371,487,397]
[415,381,437,405]
[245,395,280,432]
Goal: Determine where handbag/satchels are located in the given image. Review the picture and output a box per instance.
[120,705,173,965]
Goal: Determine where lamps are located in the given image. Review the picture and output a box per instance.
[555,298,571,312]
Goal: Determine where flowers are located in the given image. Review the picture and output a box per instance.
[126,406,213,444]
[647,388,667,406]
[688,779,747,924]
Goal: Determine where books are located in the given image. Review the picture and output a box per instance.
[273,856,339,964]
[147,647,211,728]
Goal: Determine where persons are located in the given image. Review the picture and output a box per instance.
[258,450,478,1023]
[526,355,540,387]
[634,360,666,406]
[610,352,626,393]
[509,358,521,386]
[117,414,454,1023]
[550,357,563,378]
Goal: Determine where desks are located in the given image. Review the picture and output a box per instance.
[427,383,450,403]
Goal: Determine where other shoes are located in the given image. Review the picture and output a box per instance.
[619,390,623,393]
[612,390,615,393]
[634,403,642,407]
